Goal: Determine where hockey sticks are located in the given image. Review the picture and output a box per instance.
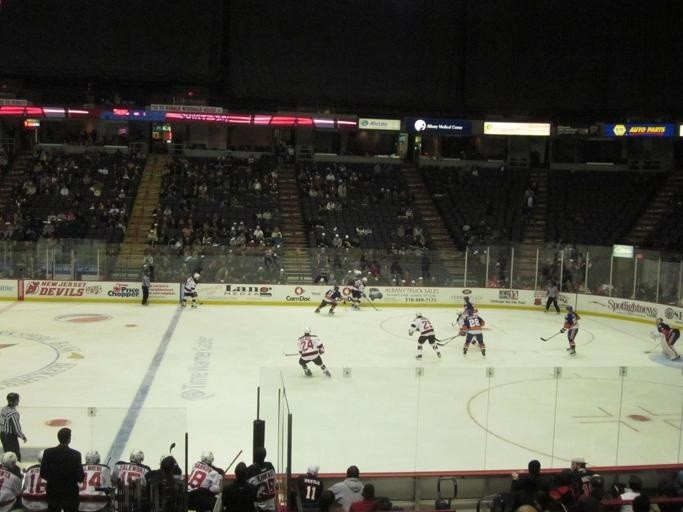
[434,333,460,346]
[643,340,661,354]
[364,297,381,311]
[540,332,561,341]
[280,351,299,357]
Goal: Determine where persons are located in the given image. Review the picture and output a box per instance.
[295,327,330,377]
[180,272,201,308]
[40,428,84,512]
[313,286,341,315]
[560,305,580,354]
[296,158,681,307]
[458,310,486,356]
[1,139,287,284]
[655,318,680,361]
[459,295,474,317]
[408,312,442,359]
[500,457,681,512]
[141,268,151,305]
[545,281,562,315]
[0,445,405,512]
[0,392,26,463]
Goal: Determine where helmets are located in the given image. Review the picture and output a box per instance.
[566,305,573,312]
[415,309,422,317]
[130,448,144,463]
[6,391,19,405]
[464,295,475,317]
[85,447,100,464]
[36,449,45,462]
[0,450,18,468]
[304,326,312,334]
[199,449,216,463]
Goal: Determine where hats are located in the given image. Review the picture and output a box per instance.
[234,460,248,476]
[570,454,588,465]
[308,462,320,476]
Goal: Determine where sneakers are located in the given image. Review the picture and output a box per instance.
[566,347,579,356]
[416,350,486,359]
[303,366,332,377]
[179,303,198,309]
[314,304,362,316]
